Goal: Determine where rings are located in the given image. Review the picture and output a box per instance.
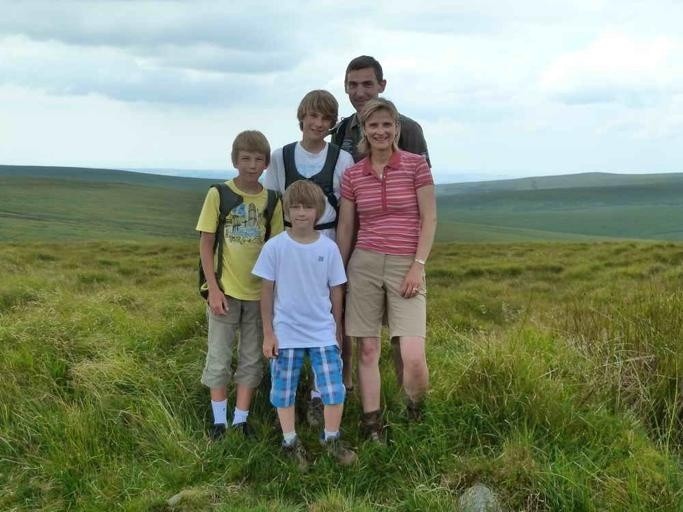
[413,287,418,291]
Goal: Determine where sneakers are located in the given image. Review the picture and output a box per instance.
[282,435,311,475]
[347,387,358,404]
[360,409,384,447]
[211,420,252,442]
[318,431,357,468]
[307,386,325,427]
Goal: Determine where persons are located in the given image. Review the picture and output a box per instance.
[250,180,359,472]
[259,89,356,428]
[329,55,431,422]
[334,98,437,450]
[194,130,285,445]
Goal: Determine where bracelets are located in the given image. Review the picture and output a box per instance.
[414,258,426,265]
